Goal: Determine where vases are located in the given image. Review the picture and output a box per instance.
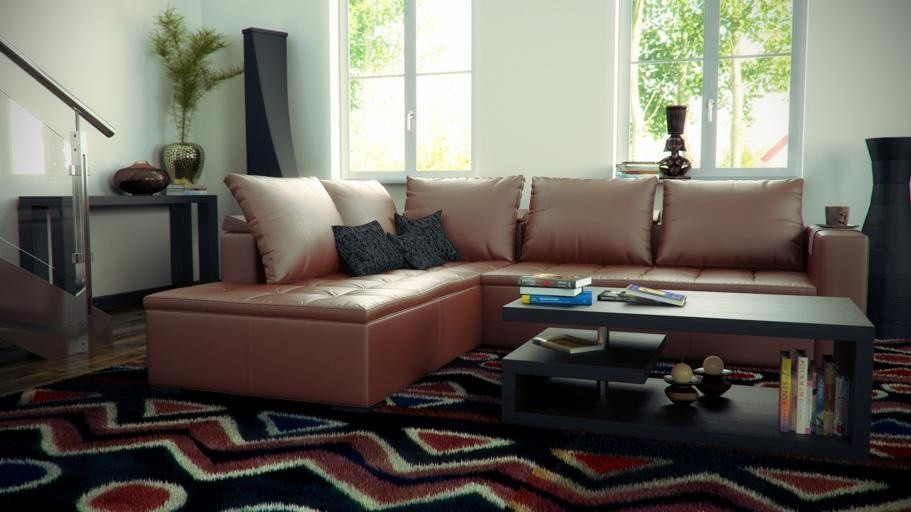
[861,137,911,339]
[114,161,170,196]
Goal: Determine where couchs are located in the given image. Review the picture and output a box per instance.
[143,202,869,408]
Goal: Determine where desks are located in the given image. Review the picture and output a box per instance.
[17,195,219,296]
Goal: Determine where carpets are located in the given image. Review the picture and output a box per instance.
[0,339,911,512]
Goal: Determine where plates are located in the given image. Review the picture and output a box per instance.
[815,222,862,229]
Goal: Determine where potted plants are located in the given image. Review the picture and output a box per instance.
[149,7,245,185]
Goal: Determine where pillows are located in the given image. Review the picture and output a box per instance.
[394,210,461,261]
[224,173,346,284]
[521,176,658,266]
[387,231,446,270]
[657,177,805,270]
[320,180,398,236]
[331,220,405,277]
[403,175,526,261]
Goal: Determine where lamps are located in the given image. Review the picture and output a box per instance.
[659,105,692,179]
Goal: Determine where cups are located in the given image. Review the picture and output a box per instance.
[824,205,851,227]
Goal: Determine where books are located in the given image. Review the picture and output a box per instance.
[517,272,686,306]
[779,349,849,438]
[532,333,604,354]
